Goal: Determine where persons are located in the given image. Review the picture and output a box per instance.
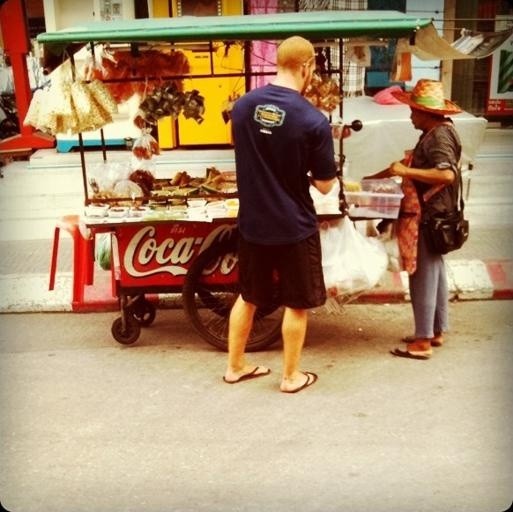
[358,77,464,360]
[219,34,338,395]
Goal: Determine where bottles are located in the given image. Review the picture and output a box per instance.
[389,37,412,83]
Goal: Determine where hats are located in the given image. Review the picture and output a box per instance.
[390,78,464,114]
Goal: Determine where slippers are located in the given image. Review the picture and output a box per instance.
[279,370,318,395]
[389,334,443,361]
[222,366,271,385]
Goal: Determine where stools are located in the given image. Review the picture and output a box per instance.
[48,215,119,303]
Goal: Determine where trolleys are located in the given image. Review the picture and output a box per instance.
[36,10,435,354]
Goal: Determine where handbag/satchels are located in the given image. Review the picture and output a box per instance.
[420,161,469,255]
